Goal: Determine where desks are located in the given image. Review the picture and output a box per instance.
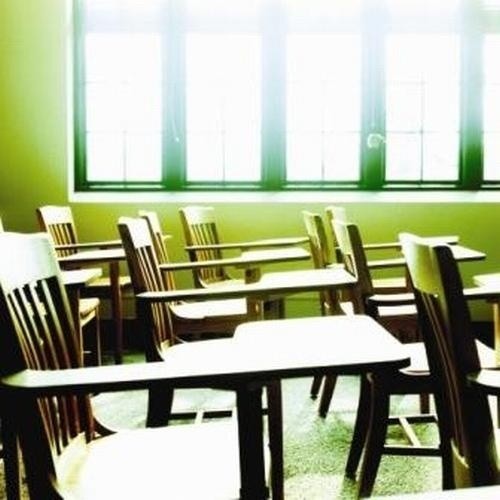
[360,235,460,249]
[183,237,310,253]
[135,269,359,321]
[366,245,487,270]
[54,234,174,251]
[61,268,102,367]
[57,247,128,365]
[1,314,412,499]
[157,247,312,285]
[366,273,500,307]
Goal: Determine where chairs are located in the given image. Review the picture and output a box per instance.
[332,218,500,500]
[398,232,499,491]
[298,206,419,421]
[78,297,101,367]
[1,226,273,500]
[178,206,245,288]
[35,205,133,298]
[139,211,264,336]
[117,218,283,499]
